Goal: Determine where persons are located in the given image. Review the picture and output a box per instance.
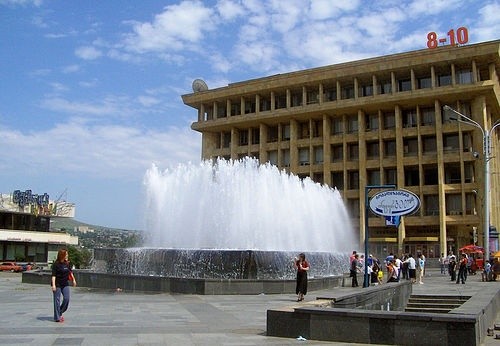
[440,251,474,284]
[50,249,76,322]
[485,258,499,281]
[293,253,310,302]
[350,250,426,288]
[26,263,32,271]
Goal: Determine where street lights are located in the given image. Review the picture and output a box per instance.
[443,104,500,270]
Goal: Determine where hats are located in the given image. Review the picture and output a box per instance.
[450,257,454,259]
[408,254,412,257]
[354,255,358,257]
[369,255,372,257]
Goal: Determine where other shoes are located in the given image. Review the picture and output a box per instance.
[298,297,304,301]
[55,317,64,322]
[419,281,424,285]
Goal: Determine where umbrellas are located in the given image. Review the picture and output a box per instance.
[459,245,484,259]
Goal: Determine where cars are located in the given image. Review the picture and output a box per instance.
[0,261,23,273]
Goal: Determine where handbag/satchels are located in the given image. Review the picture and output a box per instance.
[67,274,73,280]
